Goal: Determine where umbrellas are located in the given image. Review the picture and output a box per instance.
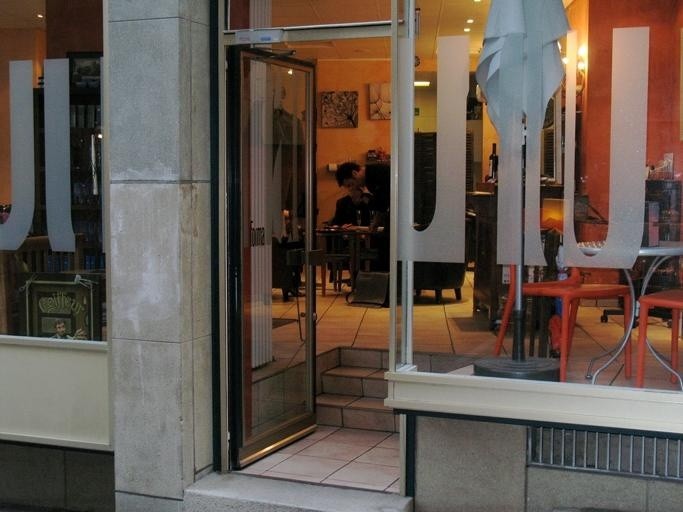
[552,233,570,318]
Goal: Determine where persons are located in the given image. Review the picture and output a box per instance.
[341,166,390,234]
[245,85,307,299]
[46,317,87,341]
[323,186,373,283]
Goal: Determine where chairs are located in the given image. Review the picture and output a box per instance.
[490,222,683,388]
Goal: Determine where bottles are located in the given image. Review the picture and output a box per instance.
[487,143,499,182]
[319,221,328,231]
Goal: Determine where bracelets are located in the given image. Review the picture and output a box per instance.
[371,222,378,227]
